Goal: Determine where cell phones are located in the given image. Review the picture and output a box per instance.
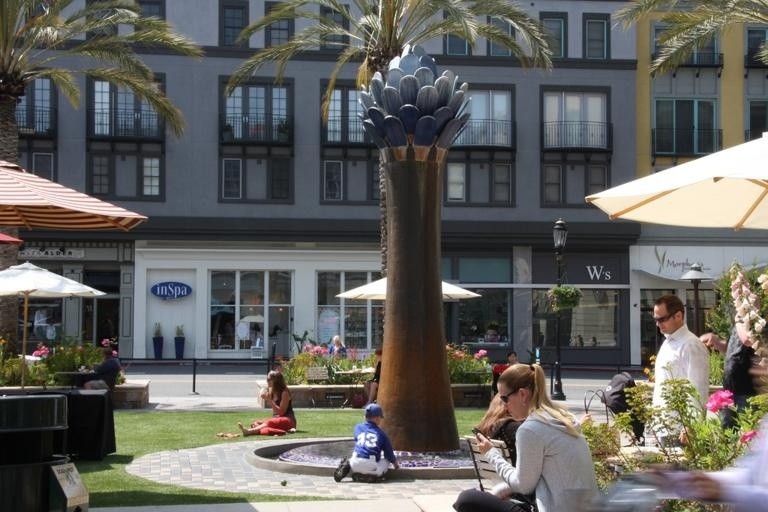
[471,427,490,442]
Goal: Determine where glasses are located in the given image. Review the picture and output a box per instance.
[653,311,677,323]
[498,390,517,400]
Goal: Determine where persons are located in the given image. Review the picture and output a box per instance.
[239,370,296,436]
[329,335,347,359]
[333,403,400,482]
[652,296,767,446]
[486,330,498,341]
[508,351,519,366]
[85,350,120,389]
[454,364,596,512]
[604,372,645,444]
[362,349,382,409]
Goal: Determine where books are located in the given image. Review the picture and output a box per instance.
[1,260,109,391]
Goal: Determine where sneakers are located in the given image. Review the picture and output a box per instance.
[333,459,351,482]
[351,472,376,484]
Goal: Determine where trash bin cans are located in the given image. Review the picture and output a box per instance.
[1,394,69,511]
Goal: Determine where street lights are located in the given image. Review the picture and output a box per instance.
[679,262,714,336]
[549,217,568,400]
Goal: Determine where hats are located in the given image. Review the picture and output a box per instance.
[364,403,384,418]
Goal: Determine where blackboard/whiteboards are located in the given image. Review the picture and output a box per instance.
[252,346,263,358]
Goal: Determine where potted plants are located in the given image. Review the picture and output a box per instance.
[151,321,186,361]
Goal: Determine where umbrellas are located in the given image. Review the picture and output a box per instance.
[585,135,768,232]
[0,232,24,245]
[333,276,483,303]
[0,157,150,235]
